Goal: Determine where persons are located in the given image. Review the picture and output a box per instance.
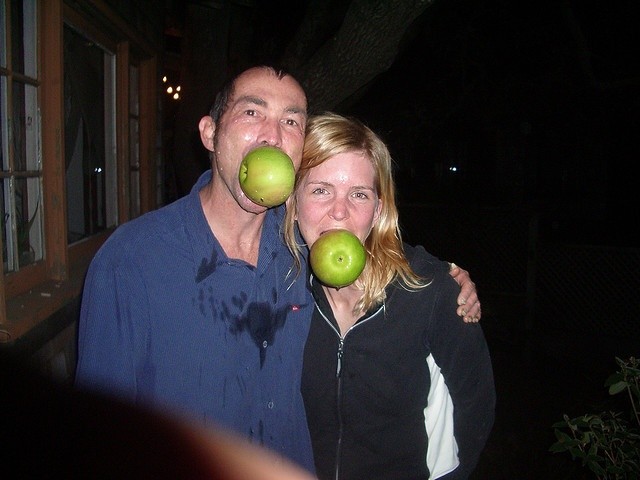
[72,64,315,480]
[283,110,499,480]
[0,340,317,480]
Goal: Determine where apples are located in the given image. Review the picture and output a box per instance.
[239,146,296,208]
[310,230,367,288]
